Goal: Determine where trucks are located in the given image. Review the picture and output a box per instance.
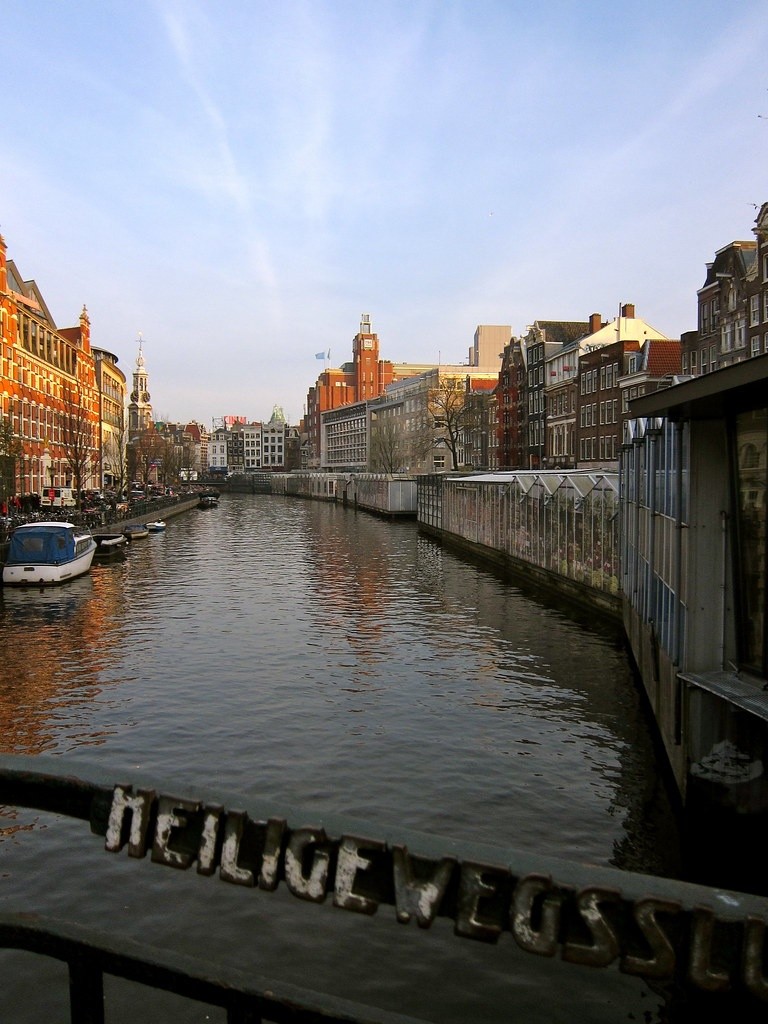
[41,485,77,511]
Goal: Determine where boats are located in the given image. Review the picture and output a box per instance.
[146,519,167,532]
[200,492,220,506]
[123,524,150,539]
[2,522,98,587]
[92,533,128,562]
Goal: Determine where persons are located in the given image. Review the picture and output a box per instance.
[153,485,156,490]
[73,490,85,505]
[2,492,39,517]
